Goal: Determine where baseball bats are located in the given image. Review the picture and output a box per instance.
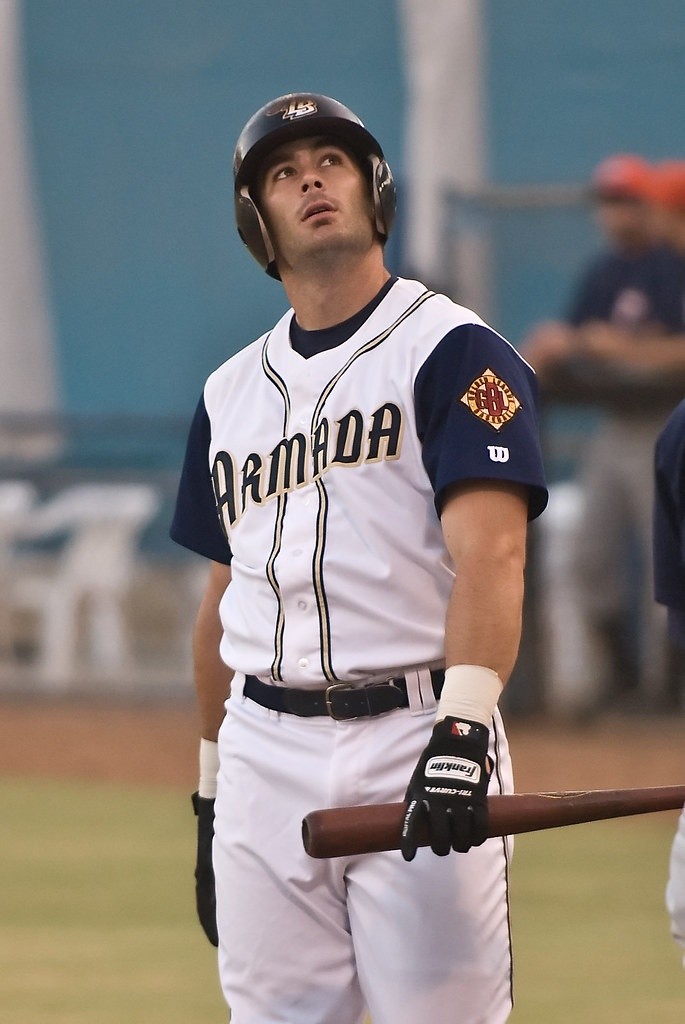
[301,785,685,859]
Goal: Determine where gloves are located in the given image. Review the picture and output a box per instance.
[399,715,494,862]
[191,790,218,947]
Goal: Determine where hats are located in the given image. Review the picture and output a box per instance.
[594,155,649,202]
[636,163,685,211]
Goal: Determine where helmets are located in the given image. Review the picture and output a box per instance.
[233,92,397,278]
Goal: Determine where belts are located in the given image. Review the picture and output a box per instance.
[243,668,445,722]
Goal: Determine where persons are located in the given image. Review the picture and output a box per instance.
[522,155,685,722]
[168,93,550,1023]
[651,398,685,963]
